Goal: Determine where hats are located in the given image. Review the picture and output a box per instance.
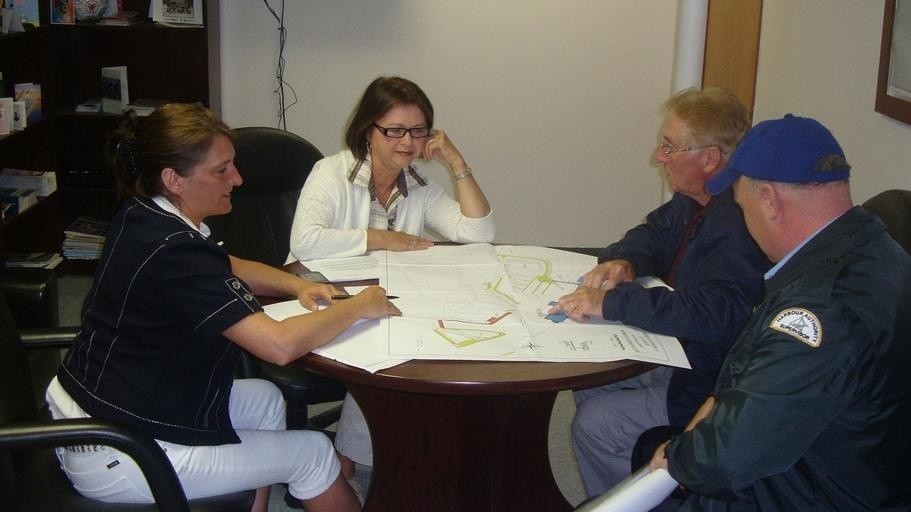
[705,113,850,196]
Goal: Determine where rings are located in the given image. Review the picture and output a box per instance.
[428,138,435,143]
[569,300,575,310]
[412,238,416,244]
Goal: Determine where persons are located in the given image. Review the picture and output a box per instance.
[284,75,497,504]
[45,103,403,512]
[547,86,779,501]
[631,113,911,511]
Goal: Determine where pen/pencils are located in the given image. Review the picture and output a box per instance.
[328,294,400,300]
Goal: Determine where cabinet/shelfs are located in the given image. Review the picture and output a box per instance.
[0,0,222,276]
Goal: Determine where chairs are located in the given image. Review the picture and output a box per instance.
[0,298,253,511]
[201,127,347,507]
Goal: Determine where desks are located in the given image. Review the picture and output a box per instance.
[256,242,687,511]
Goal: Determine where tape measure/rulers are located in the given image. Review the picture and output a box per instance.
[545,275,590,323]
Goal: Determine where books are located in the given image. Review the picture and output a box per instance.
[1,83,41,135]
[62,217,111,260]
[1,2,119,35]
[5,250,62,269]
[1,167,57,220]
[75,65,155,117]
[149,2,204,24]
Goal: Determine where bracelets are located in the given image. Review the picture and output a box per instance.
[453,166,473,182]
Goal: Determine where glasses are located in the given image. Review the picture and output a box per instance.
[374,123,430,138]
[655,137,728,156]
